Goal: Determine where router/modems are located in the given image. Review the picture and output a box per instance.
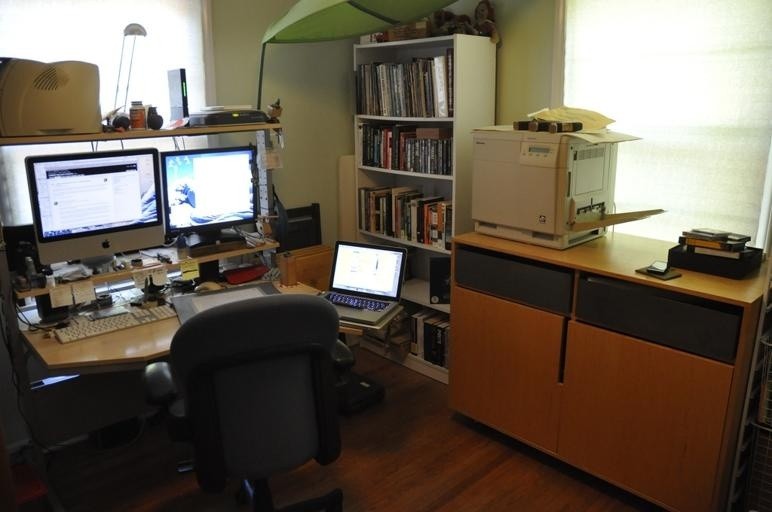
[205,111,269,124]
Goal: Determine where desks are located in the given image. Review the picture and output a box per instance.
[8,278,327,511]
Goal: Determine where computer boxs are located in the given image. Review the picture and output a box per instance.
[0,56,103,138]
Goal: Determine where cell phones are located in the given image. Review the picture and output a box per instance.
[647,260,670,275]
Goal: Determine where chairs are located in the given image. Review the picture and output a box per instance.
[139,292,355,512]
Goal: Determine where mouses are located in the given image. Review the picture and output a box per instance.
[194,280,222,292]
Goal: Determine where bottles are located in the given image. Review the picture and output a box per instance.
[23,255,55,288]
[129,101,146,129]
[177,236,186,259]
[100,240,117,272]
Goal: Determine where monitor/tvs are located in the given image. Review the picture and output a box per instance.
[159,147,262,257]
[21,148,164,276]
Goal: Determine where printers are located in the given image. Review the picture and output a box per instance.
[470,124,668,251]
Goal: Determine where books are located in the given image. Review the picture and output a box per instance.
[678,227,752,260]
[407,307,449,371]
[354,47,453,253]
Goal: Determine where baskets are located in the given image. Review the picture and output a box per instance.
[742,429,772,511]
[756,336,771,428]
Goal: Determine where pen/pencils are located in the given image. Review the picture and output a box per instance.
[144,277,148,304]
[149,275,153,284]
[89,315,95,321]
[71,317,79,325]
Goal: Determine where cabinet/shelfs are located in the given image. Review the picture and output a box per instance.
[444,225,770,511]
[0,122,284,323]
[350,34,498,387]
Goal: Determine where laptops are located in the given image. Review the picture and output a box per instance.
[316,241,409,327]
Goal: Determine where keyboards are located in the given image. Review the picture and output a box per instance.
[53,302,178,345]
